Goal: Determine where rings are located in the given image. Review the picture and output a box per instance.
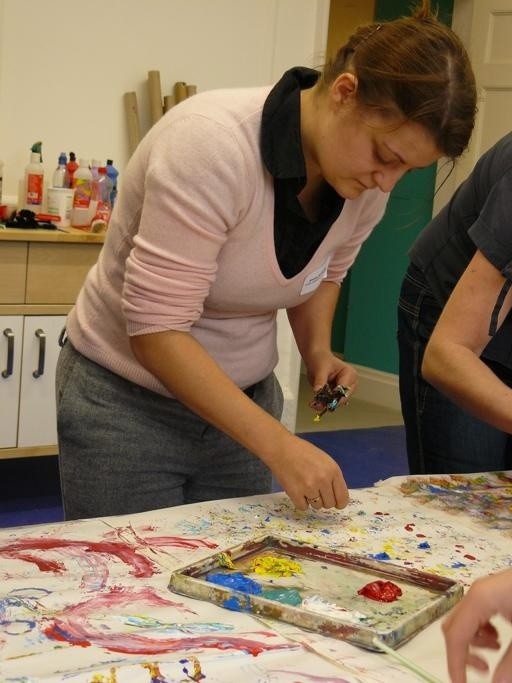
[306,495,322,504]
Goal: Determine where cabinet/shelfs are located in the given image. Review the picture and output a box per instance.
[1,227,108,460]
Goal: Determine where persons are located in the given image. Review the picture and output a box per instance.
[53,0,478,536]
[393,126,510,477]
[437,567,512,683]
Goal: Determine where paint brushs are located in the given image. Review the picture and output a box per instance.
[372,638,440,683]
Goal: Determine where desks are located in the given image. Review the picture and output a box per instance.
[0,469,510,683]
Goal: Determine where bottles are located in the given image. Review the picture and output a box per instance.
[45,150,120,231]
